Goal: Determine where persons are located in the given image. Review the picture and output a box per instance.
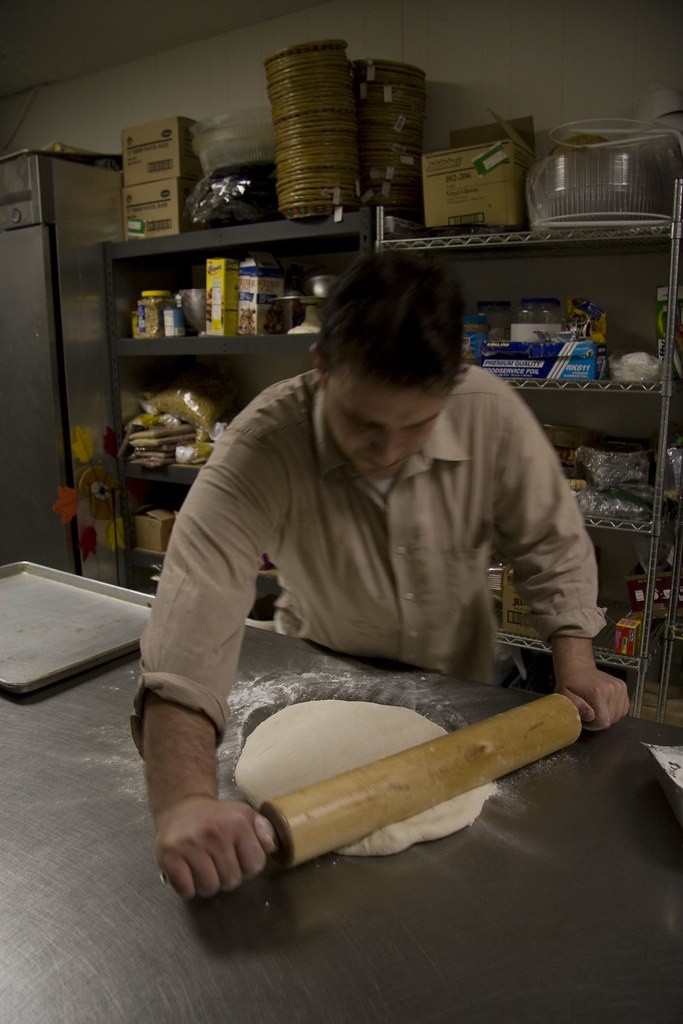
[129,249,631,899]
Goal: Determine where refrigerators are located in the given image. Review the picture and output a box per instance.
[0,147,127,591]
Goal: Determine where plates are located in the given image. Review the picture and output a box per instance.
[350,59,424,222]
[261,39,357,219]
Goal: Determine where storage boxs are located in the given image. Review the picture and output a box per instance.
[132,499,183,552]
[421,106,537,225]
[479,339,607,380]
[615,610,644,656]
[624,560,683,618]
[502,562,540,639]
[122,178,212,243]
[122,116,204,186]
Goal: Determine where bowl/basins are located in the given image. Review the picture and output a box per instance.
[179,288,206,335]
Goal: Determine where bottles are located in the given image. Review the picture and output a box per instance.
[476,301,511,341]
[461,315,489,368]
[518,297,561,343]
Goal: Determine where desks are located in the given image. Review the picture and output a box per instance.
[0,625,683,1024]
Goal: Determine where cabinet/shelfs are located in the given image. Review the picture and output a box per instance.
[102,204,432,626]
[376,178,683,722]
